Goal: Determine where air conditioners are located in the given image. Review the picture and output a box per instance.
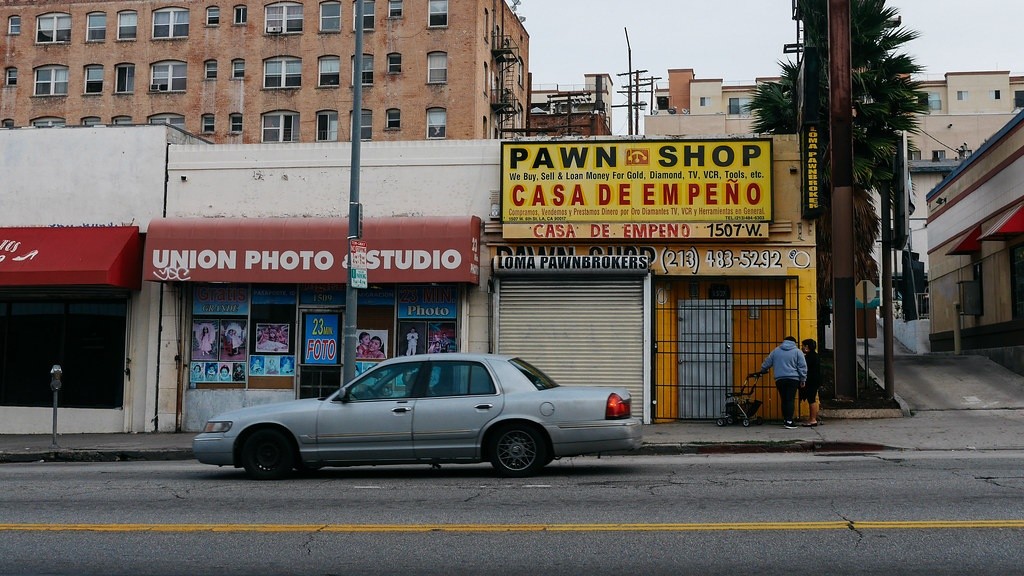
[150,84,159,92]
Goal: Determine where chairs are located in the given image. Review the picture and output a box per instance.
[404,371,431,397]
[432,367,453,396]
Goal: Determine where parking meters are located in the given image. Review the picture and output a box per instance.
[50,364,63,449]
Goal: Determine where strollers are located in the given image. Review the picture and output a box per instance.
[715,371,765,427]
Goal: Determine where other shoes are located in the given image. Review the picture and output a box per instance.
[802,420,817,427]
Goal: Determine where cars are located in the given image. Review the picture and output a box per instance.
[191,352,644,479]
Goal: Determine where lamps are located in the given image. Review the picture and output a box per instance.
[936,198,947,205]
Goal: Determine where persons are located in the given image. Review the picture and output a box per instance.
[250,356,295,376]
[191,363,245,382]
[199,326,212,356]
[761,336,807,429]
[406,327,418,356]
[356,332,386,358]
[227,329,240,355]
[798,339,821,427]
[431,332,447,353]
[257,325,288,346]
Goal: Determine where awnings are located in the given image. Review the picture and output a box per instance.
[141,215,481,286]
[0,226,142,290]
[945,224,982,255]
[975,203,1024,241]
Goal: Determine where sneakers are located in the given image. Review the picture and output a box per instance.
[783,419,786,427]
[785,421,797,428]
